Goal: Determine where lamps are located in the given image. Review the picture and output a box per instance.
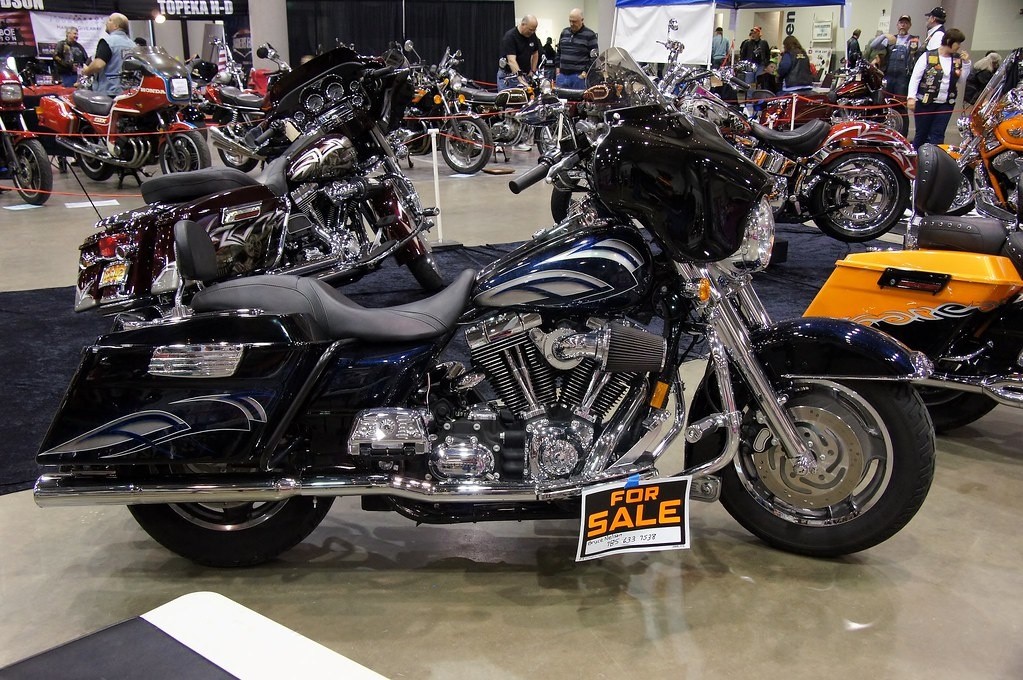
[151,9,165,24]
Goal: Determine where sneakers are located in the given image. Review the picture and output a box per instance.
[512,143,532,151]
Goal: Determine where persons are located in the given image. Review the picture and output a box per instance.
[53,27,92,88]
[82,13,148,94]
[300,53,314,66]
[543,8,600,100]
[497,14,539,92]
[876,7,1002,151]
[712,27,732,71]
[847,28,862,69]
[740,26,816,95]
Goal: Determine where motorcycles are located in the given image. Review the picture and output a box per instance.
[30,95,936,565]
[0,42,1023,239]
[737,138,1022,431]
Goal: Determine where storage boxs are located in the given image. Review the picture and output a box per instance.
[800,249,1023,329]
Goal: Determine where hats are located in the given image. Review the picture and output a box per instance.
[899,14,911,21]
[751,26,760,32]
[924,6,947,19]
